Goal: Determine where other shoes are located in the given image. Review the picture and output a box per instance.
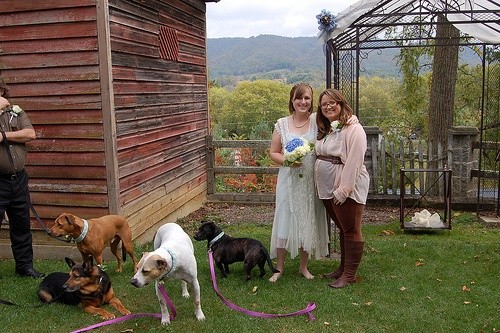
[16,266,45,278]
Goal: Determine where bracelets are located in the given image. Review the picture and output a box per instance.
[1,131,8,142]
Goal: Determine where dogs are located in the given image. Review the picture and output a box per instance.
[193,219,280,283]
[129,222,206,326]
[48,211,139,273]
[38,251,130,321]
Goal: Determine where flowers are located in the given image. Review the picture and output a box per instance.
[315,9,337,55]
[282,137,315,178]
[330,120,343,133]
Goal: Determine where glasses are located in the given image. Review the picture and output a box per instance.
[319,101,339,108]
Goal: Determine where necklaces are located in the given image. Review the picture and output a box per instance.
[292,115,309,128]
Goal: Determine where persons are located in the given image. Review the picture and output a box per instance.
[268,82,359,282]
[0,75,46,278]
[313,89,371,289]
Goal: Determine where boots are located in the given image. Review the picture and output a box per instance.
[322,241,345,279]
[328,241,365,288]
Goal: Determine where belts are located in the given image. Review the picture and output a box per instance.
[0,168,25,181]
